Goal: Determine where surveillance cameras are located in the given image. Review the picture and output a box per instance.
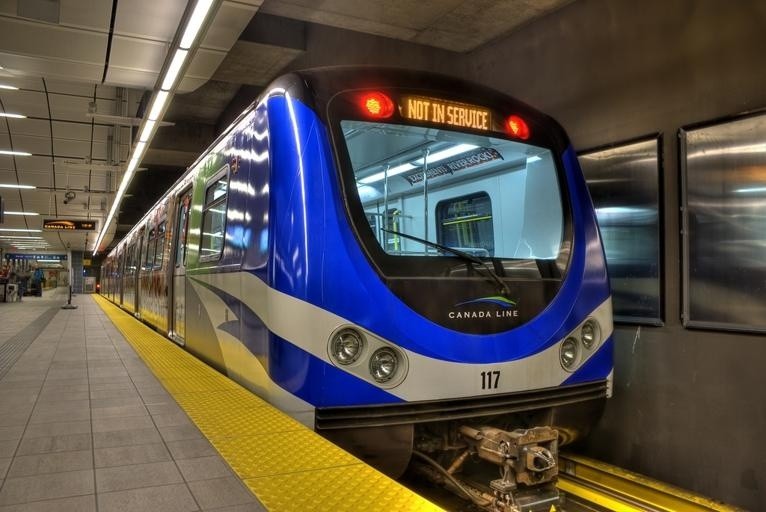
[64,198,71,204]
[67,243,70,248]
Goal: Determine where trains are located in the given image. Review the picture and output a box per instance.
[96,62,622,511]
